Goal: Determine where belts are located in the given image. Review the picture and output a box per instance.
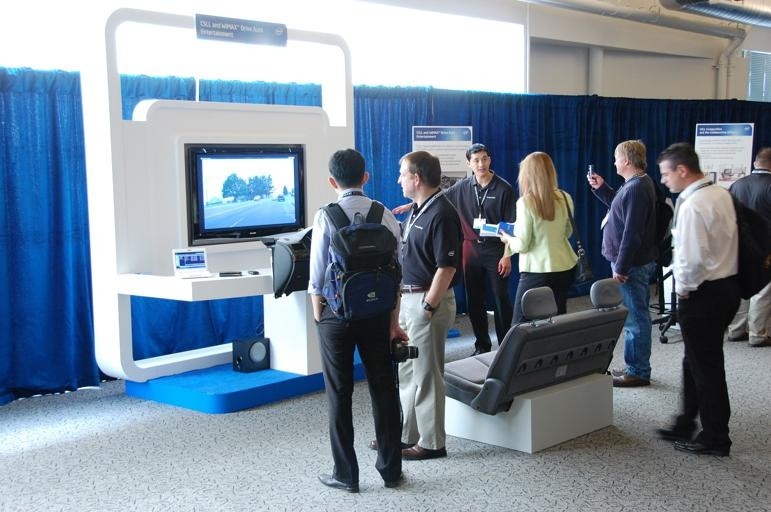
[472,239,497,243]
[401,286,428,294]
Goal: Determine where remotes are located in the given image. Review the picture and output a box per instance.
[219,272,242,277]
[248,271,259,275]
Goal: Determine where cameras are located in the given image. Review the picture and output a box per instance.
[392,337,419,363]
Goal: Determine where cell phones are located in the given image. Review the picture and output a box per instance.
[588,164,595,180]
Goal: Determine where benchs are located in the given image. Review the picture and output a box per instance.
[440,277,632,458]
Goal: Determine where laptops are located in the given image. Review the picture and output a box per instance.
[173,247,213,279]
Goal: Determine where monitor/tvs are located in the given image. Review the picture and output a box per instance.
[191,148,304,239]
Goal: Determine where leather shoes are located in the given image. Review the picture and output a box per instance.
[611,332,770,455]
[320,437,447,493]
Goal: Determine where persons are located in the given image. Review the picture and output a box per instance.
[654,140,742,458]
[725,146,771,348]
[391,143,515,356]
[497,152,577,328]
[396,151,455,464]
[310,148,408,491]
[584,138,661,391]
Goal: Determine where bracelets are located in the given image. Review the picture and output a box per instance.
[422,301,436,312]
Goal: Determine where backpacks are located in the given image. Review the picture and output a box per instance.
[321,203,398,322]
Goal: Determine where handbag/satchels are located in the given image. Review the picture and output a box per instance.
[575,248,593,287]
[727,189,770,299]
[653,174,675,264]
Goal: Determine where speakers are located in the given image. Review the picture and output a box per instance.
[233,335,270,373]
